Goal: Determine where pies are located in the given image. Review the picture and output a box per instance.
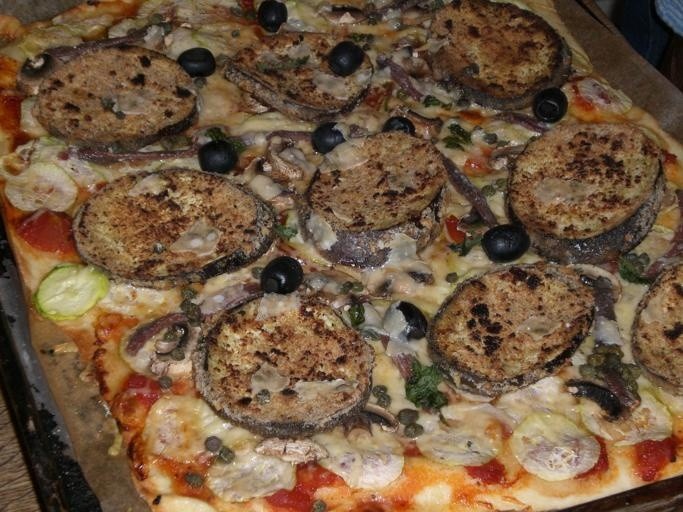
[2,0,683,512]
